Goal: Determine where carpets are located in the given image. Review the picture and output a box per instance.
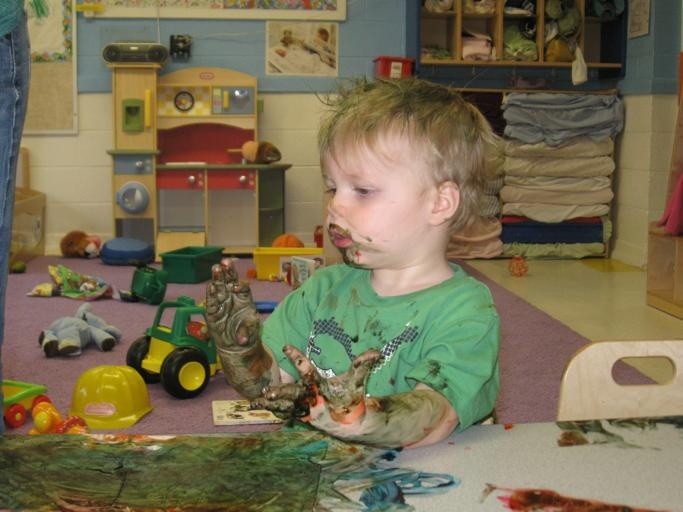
[0,253,658,433]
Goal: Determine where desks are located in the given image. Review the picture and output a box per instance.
[1,414,682,508]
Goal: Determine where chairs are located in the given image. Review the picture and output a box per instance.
[558,340,682,422]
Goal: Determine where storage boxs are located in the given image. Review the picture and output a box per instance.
[371,54,413,83]
[251,247,325,281]
[158,246,223,283]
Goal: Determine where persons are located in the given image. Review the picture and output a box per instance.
[202,74,506,451]
[1,0,30,438]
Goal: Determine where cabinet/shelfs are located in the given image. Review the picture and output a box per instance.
[645,221,682,319]
[405,0,625,96]
[108,64,262,249]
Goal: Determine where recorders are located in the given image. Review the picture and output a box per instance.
[101,41,168,63]
